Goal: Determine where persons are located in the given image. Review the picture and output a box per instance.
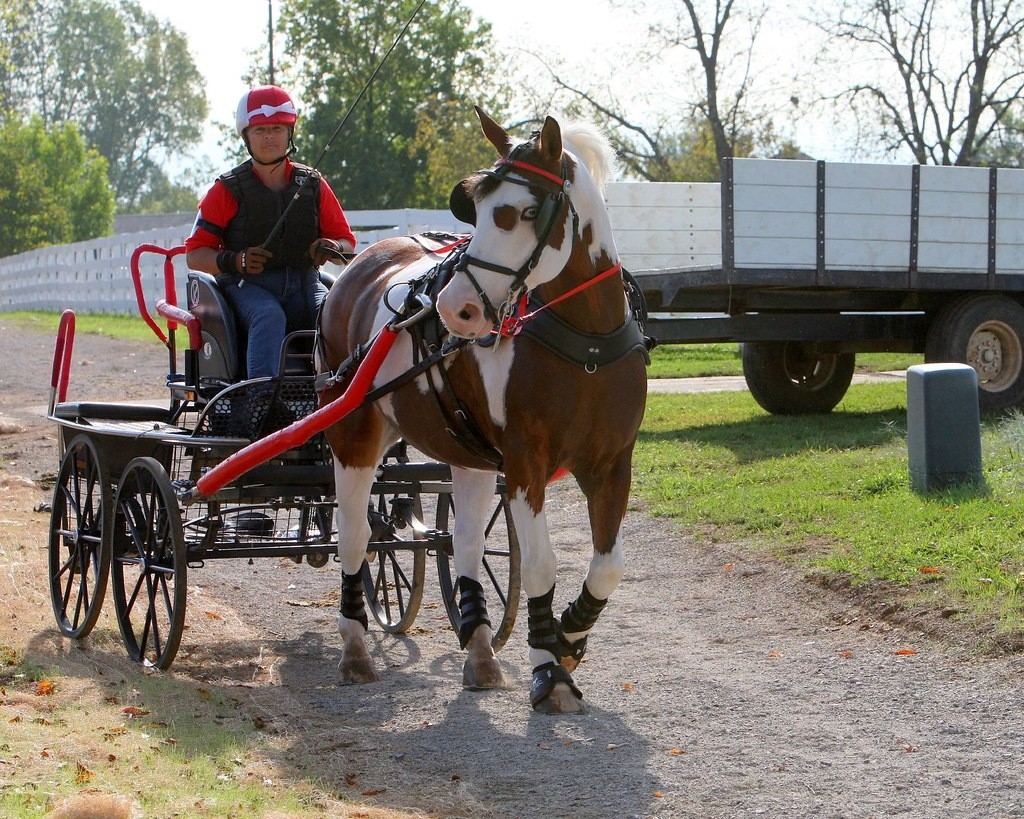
[184,84,355,433]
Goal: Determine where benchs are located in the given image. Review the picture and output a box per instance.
[187,271,337,405]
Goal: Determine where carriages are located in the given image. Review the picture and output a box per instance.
[45,103,658,714]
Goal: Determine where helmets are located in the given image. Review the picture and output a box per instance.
[236,85,299,135]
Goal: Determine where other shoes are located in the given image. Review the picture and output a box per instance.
[251,396,292,441]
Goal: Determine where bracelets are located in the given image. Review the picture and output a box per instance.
[334,239,343,254]
[216,248,237,278]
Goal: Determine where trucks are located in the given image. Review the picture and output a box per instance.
[599,156,1024,420]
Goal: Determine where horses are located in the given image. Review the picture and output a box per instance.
[310,105,648,717]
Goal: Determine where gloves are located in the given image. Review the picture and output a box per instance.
[216,245,272,275]
[309,238,343,265]
